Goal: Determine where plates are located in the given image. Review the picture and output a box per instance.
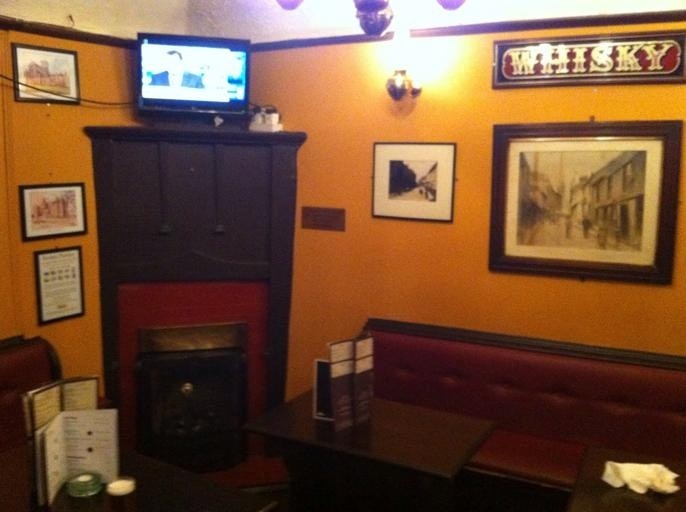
[106,478,136,496]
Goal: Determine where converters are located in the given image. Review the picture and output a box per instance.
[265,113,279,124]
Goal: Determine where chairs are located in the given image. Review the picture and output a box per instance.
[1,328,72,509]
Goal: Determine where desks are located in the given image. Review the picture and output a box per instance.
[39,443,260,511]
[565,441,685,512]
[241,381,495,509]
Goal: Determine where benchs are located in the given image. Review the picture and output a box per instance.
[345,315,685,508]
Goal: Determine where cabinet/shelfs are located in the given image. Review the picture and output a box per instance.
[78,120,311,286]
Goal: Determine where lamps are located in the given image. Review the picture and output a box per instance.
[376,40,421,103]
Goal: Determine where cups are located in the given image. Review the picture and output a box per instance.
[66,471,101,498]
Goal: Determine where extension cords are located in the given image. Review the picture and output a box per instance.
[248,121,284,133]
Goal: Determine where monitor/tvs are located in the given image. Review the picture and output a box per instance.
[134,32,250,117]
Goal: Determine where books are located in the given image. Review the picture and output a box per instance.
[21,374,98,439]
[34,407,119,505]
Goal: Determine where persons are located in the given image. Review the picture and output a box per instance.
[559,209,608,247]
[149,50,203,89]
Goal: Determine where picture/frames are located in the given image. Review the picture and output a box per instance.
[372,139,457,224]
[31,246,85,326]
[489,26,686,92]
[16,180,89,243]
[10,42,81,103]
[487,117,684,288]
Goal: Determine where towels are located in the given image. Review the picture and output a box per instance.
[601,461,682,497]
